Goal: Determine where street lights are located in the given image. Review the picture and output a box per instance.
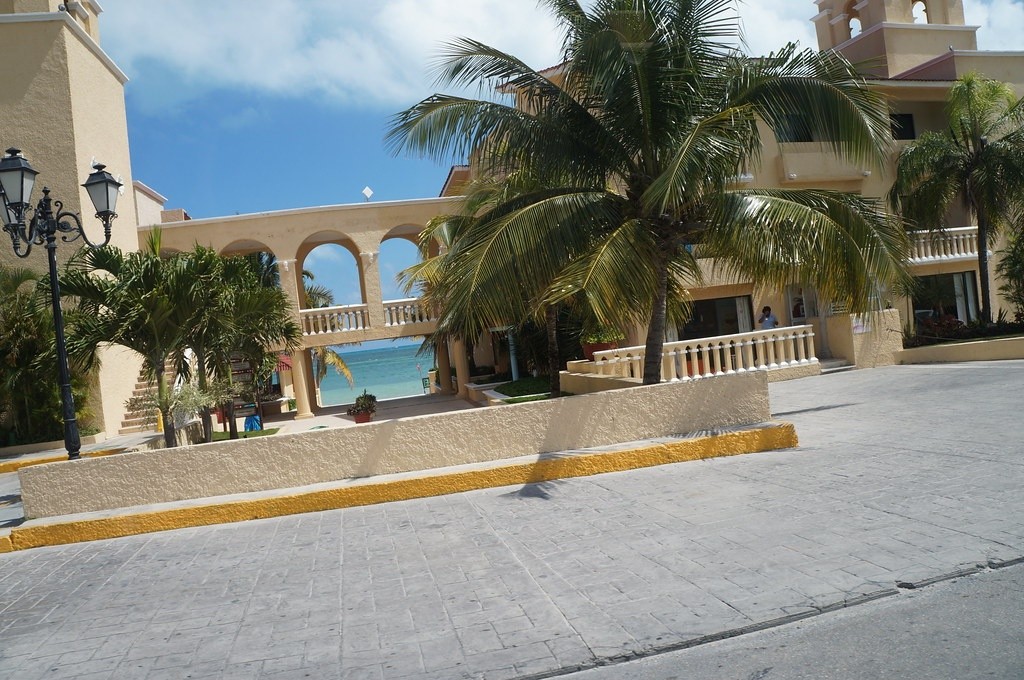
[0,144,124,458]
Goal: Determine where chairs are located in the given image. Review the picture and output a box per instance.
[422,377,430,394]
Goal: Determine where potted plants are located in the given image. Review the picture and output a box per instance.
[580,317,626,362]
[347,389,379,424]
[261,393,283,416]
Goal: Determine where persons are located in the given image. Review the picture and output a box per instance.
[756,306,779,331]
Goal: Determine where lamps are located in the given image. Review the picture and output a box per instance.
[359,250,380,264]
[276,258,298,272]
